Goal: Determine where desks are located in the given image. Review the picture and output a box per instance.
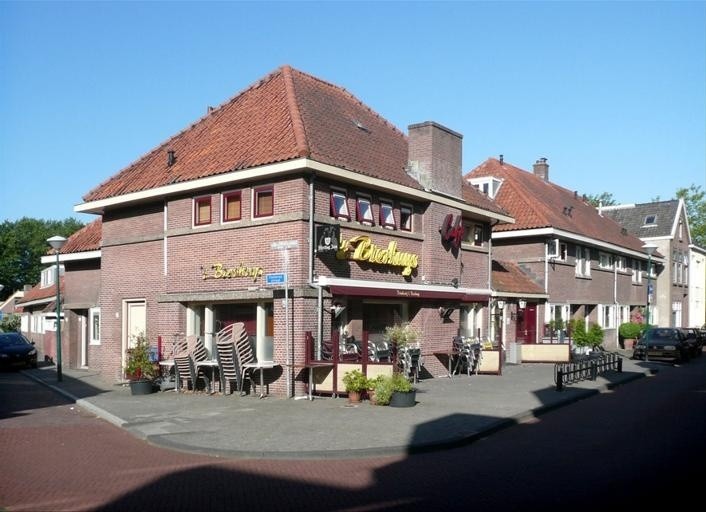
[192,361,220,393]
[159,360,177,391]
[241,362,280,398]
[288,362,334,401]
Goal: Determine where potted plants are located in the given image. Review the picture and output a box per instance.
[125,338,157,395]
[364,374,394,405]
[345,367,365,403]
[391,371,418,407]
[618,320,642,349]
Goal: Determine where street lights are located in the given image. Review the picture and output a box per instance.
[46,235,68,382]
[641,244,659,362]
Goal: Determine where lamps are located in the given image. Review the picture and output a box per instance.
[490,298,506,316]
[511,299,528,318]
[451,278,458,287]
[322,304,346,318]
[439,306,454,319]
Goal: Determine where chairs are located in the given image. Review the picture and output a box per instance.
[215,322,256,396]
[451,335,481,377]
[321,334,392,362]
[172,334,209,392]
[395,342,423,384]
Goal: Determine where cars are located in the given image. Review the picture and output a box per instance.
[0,332,38,370]
[633,323,706,366]
